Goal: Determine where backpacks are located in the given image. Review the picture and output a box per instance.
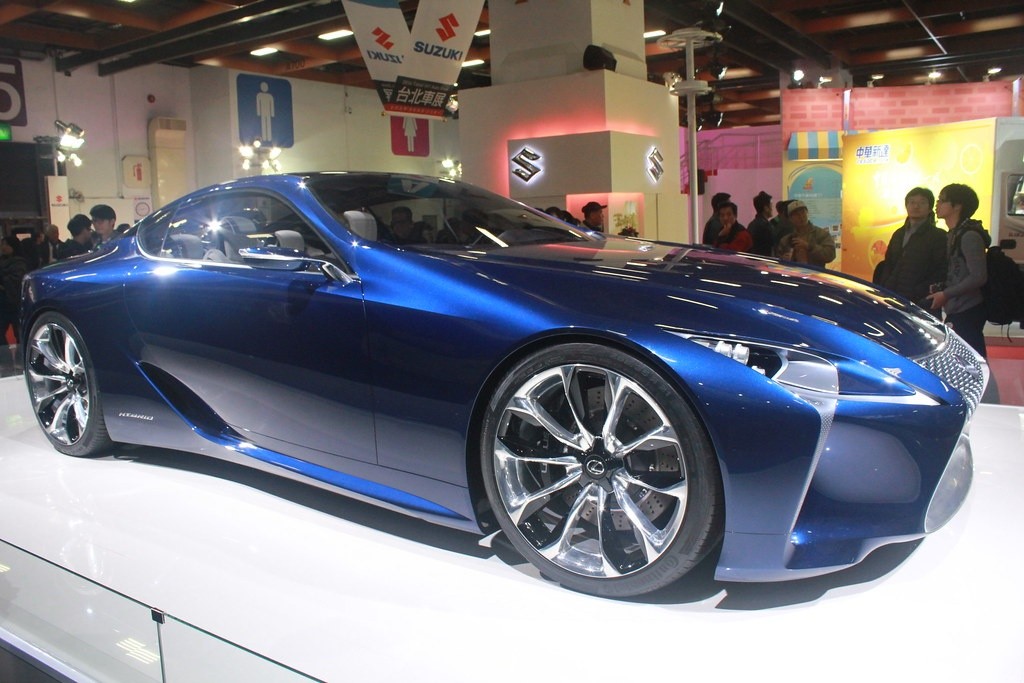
[955,228,1024,325]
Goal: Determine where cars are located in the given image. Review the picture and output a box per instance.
[18,172,992,600]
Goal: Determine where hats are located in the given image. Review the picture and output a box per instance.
[787,201,808,214]
[582,202,608,213]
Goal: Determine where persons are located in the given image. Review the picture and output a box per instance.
[0,205,131,370]
[388,205,501,245]
[703,192,836,267]
[536,202,607,232]
[927,183,999,403]
[885,187,947,322]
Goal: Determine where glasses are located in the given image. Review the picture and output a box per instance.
[87,227,93,233]
[936,198,951,205]
